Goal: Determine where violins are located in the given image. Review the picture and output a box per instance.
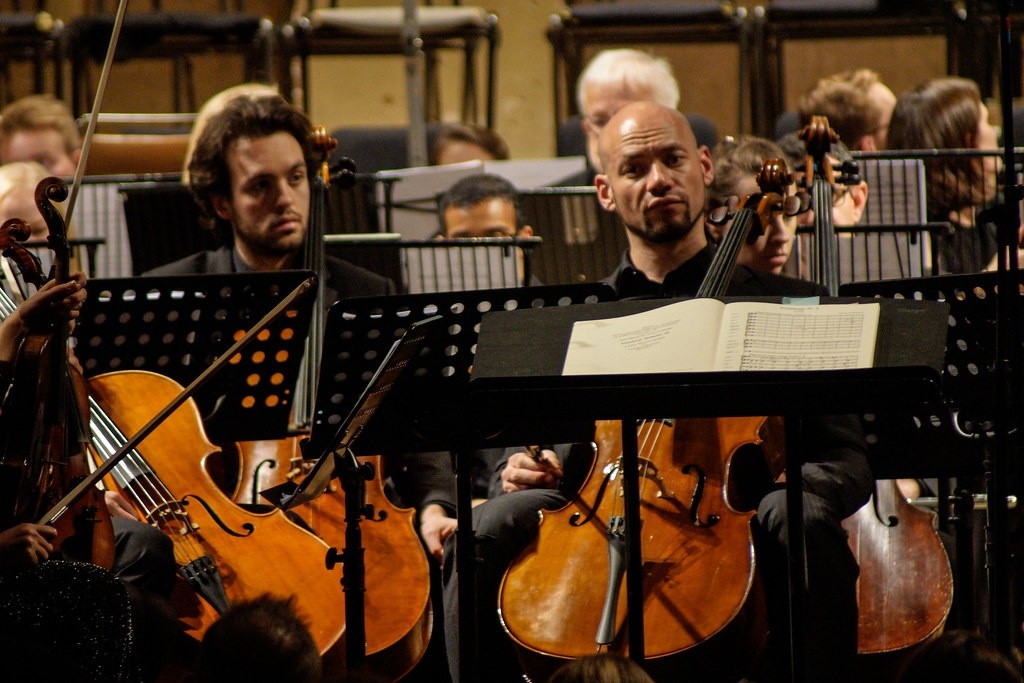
[0,177,118,574]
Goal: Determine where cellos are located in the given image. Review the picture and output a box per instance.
[225,122,436,683]
[792,113,955,663]
[495,155,803,666]
[0,210,351,666]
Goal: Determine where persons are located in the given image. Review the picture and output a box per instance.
[391,169,593,683]
[438,101,876,683]
[187,594,323,683]
[423,120,512,169]
[135,86,467,683]
[535,48,1022,501]
[1,90,175,682]
